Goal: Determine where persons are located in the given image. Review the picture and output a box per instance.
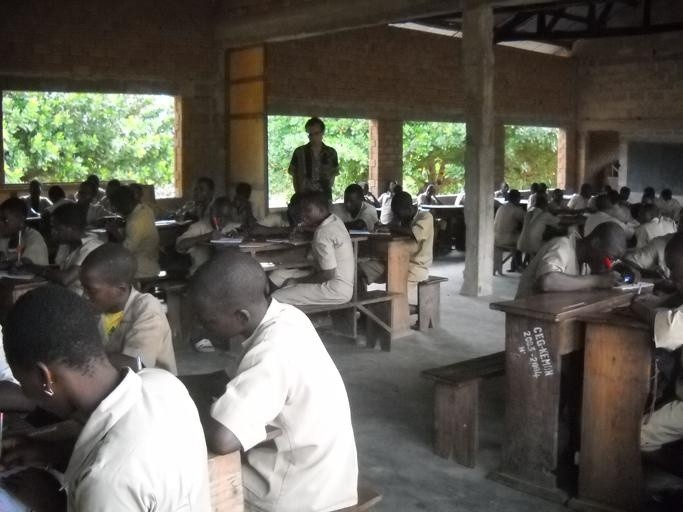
[493,180,682,283]
[0,174,161,297]
[188,248,361,512]
[169,176,258,277]
[268,178,467,327]
[288,117,340,204]
[78,242,178,376]
[514,221,627,382]
[629,232,683,453]
[0,286,212,512]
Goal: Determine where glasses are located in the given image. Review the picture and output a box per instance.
[307,130,323,138]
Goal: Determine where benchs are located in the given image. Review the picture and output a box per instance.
[154,282,189,352]
[295,291,403,352]
[493,245,537,277]
[414,276,448,330]
[437,192,529,205]
[420,350,505,469]
[333,481,384,512]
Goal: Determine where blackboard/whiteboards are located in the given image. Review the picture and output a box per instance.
[626,142,683,197]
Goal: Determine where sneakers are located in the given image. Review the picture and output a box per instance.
[194,338,216,354]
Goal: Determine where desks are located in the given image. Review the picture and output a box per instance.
[349,232,412,293]
[371,205,384,214]
[194,233,313,267]
[489,274,654,504]
[422,204,466,252]
[568,289,682,511]
[0,267,49,307]
[89,220,182,259]
[0,425,283,512]
[24,214,46,224]
[494,197,535,207]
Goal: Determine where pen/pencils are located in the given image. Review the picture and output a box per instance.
[605,257,614,271]
[17,232,21,265]
[213,216,220,232]
